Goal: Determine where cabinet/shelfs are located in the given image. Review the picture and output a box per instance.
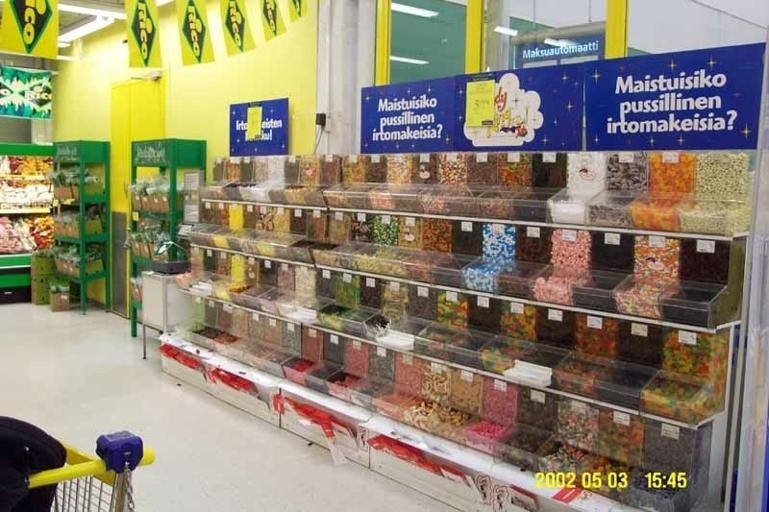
[53,140,111,315]
[141,271,192,359]
[0,142,56,289]
[132,138,206,337]
[157,149,761,512]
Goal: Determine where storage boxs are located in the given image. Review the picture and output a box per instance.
[30,256,52,305]
[49,291,69,311]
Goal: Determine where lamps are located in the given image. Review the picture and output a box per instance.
[57,15,114,48]
[57,1,129,20]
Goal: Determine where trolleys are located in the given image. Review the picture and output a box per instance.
[0,417,160,507]
